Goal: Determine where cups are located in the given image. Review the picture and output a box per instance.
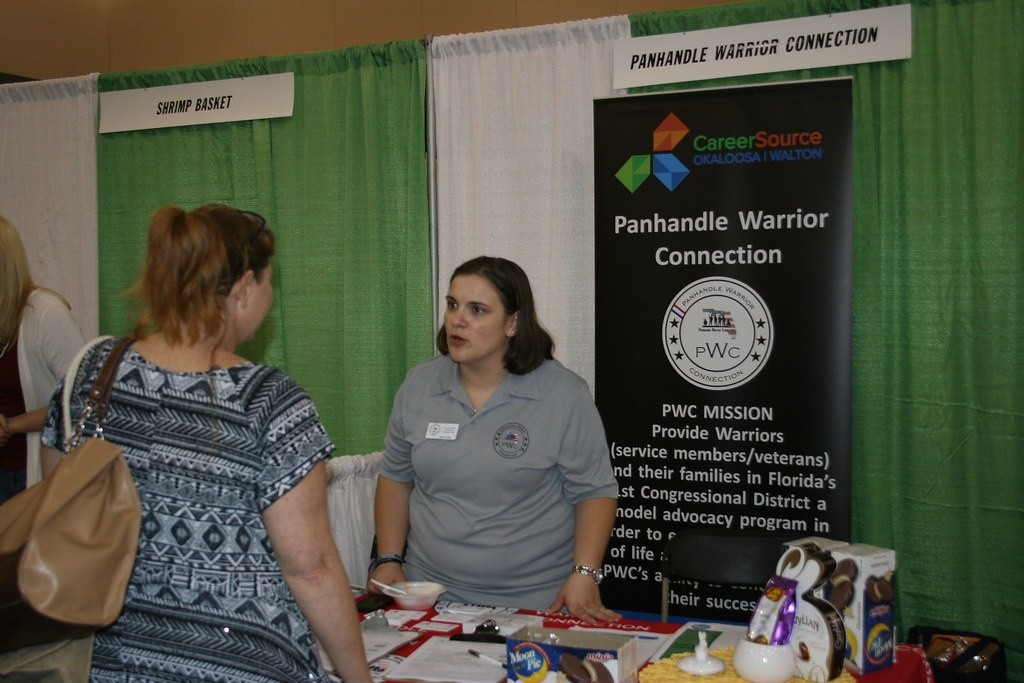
[732,634,795,683]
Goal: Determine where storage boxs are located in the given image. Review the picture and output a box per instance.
[781,536,850,599]
[506,625,638,683]
[825,543,898,675]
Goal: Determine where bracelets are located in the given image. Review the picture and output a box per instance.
[369,554,401,567]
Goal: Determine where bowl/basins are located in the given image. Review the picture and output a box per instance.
[382,581,449,610]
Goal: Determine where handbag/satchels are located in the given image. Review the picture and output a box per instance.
[0,332,143,650]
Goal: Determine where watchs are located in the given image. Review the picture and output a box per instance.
[572,563,602,583]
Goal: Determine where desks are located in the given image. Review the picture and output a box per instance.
[355,594,934,683]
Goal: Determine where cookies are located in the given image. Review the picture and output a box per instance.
[867,576,893,602]
[582,659,614,683]
[559,653,591,683]
[830,576,853,608]
[833,559,856,581]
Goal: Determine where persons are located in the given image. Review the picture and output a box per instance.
[40,202,374,682]
[366,256,622,625]
[0,216,90,682]
